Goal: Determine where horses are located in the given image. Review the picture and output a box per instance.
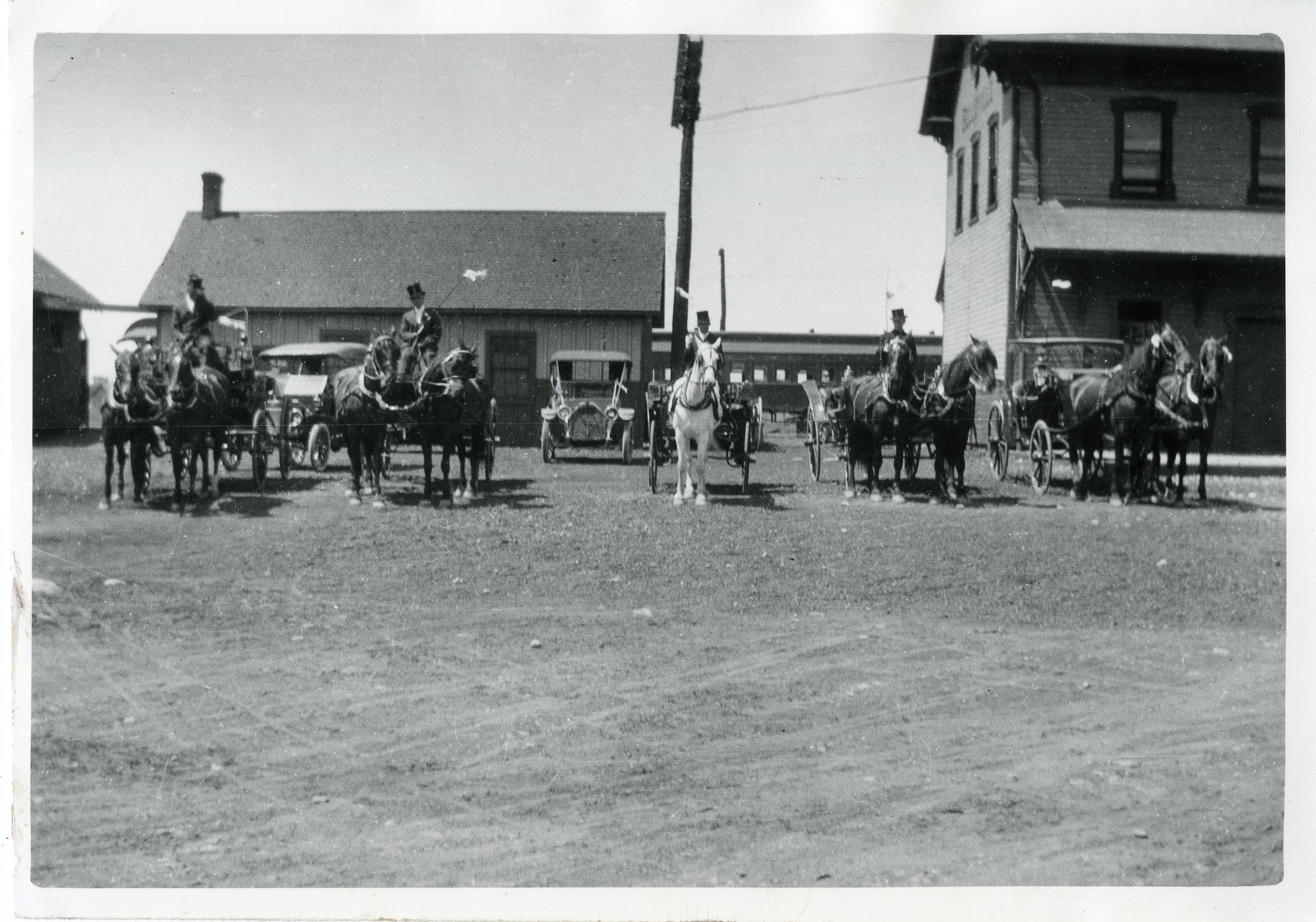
[663,339,725,510]
[99,334,237,507]
[842,330,999,503]
[1065,323,1232,508]
[330,325,501,510]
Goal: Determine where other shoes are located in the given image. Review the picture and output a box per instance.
[720,428,729,437]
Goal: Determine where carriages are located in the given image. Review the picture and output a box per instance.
[643,333,757,505]
[801,326,1000,509]
[983,322,1235,505]
[98,311,290,510]
[328,326,499,512]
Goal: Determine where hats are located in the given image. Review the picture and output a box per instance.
[186,274,203,285]
[407,283,426,299]
[890,309,907,321]
[697,311,710,324]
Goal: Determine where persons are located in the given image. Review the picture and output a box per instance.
[395,283,442,385]
[878,309,916,372]
[683,311,732,412]
[171,274,232,451]
[1021,361,1065,432]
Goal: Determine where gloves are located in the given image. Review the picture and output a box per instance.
[685,334,693,349]
[883,341,890,352]
[411,332,418,343]
[192,288,205,304]
[416,341,424,352]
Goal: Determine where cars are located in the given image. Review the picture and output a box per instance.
[220,342,372,467]
[540,349,634,464]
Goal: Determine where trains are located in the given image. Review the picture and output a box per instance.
[652,328,943,412]
